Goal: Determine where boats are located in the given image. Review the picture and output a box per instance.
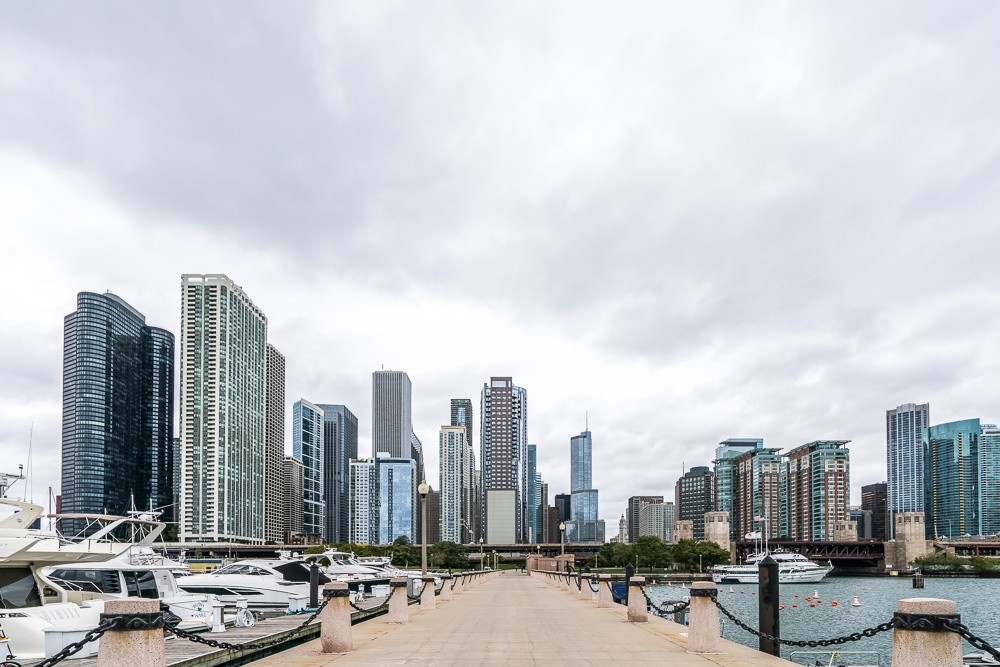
[711,508,836,585]
[0,421,431,667]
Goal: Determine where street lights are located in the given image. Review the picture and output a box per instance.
[558,521,565,555]
[699,554,703,573]
[486,554,490,567]
[417,479,430,576]
[635,555,639,575]
[480,537,483,571]
[537,545,541,570]
